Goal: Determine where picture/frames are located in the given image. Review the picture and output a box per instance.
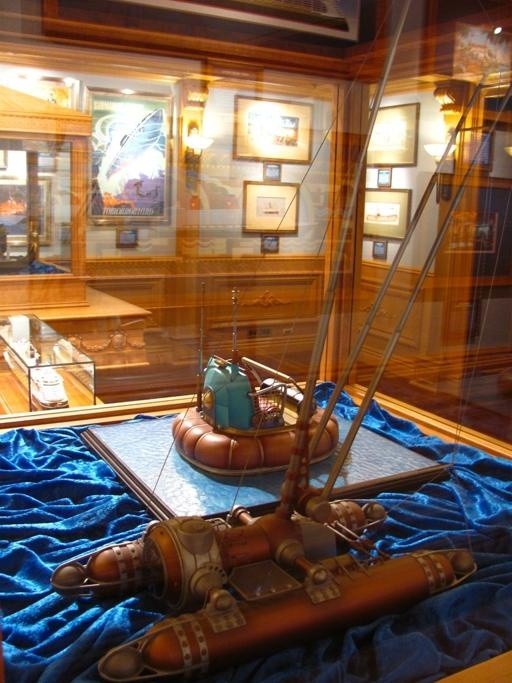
[442,209,500,254]
[0,175,53,248]
[363,187,412,241]
[233,93,315,165]
[86,84,175,227]
[366,101,421,168]
[242,180,300,234]
[454,126,497,173]
